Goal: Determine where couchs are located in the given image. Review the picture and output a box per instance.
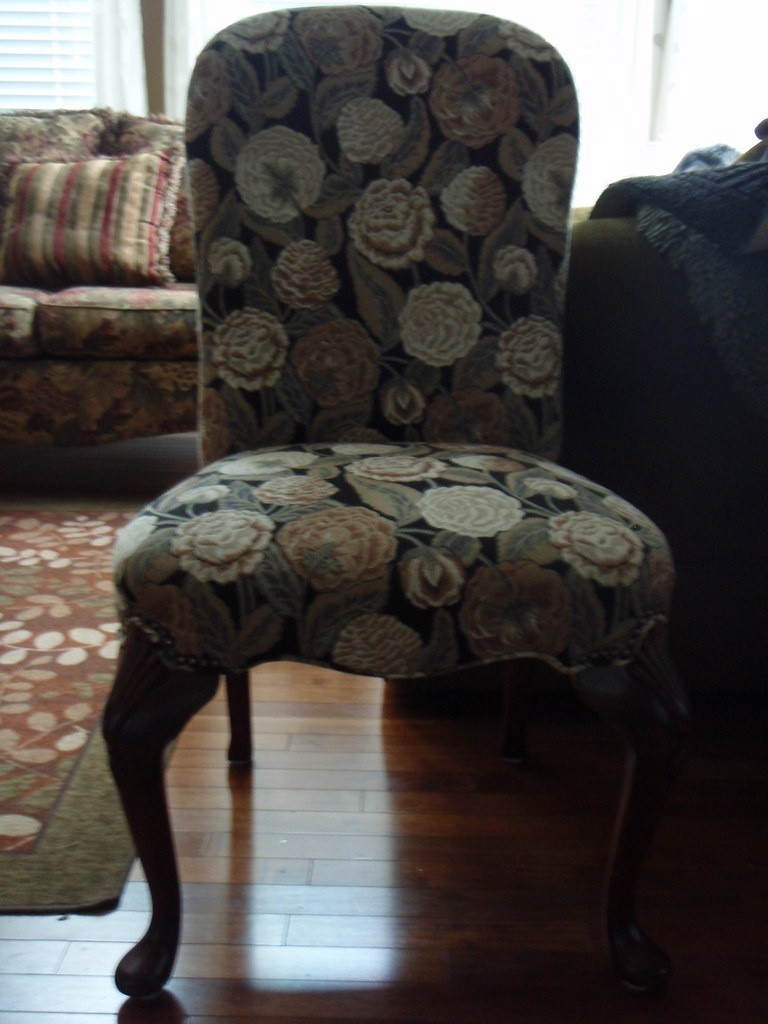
[0,105,199,492]
[400,184,768,698]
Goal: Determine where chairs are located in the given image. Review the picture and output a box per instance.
[98,4,679,998]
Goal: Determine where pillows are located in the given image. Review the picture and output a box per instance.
[0,138,186,290]
[112,111,196,286]
[0,107,111,242]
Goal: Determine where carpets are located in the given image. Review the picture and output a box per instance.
[0,492,179,917]
